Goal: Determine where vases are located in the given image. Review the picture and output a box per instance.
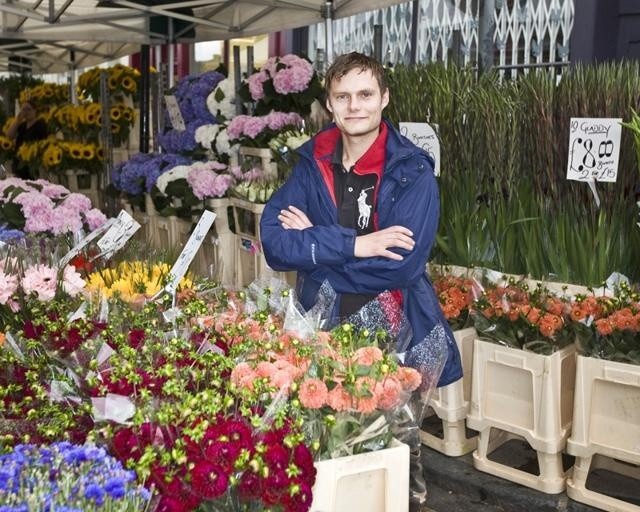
[473,339,575,496]
[565,355,640,512]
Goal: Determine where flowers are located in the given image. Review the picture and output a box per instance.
[226,112,301,145]
[243,54,313,100]
[427,268,477,321]
[0,173,317,512]
[77,64,141,99]
[231,327,422,416]
[109,151,273,200]
[571,273,639,364]
[2,83,137,165]
[468,276,572,353]
[155,70,236,159]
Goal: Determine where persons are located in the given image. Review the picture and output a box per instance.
[257,50,465,511]
